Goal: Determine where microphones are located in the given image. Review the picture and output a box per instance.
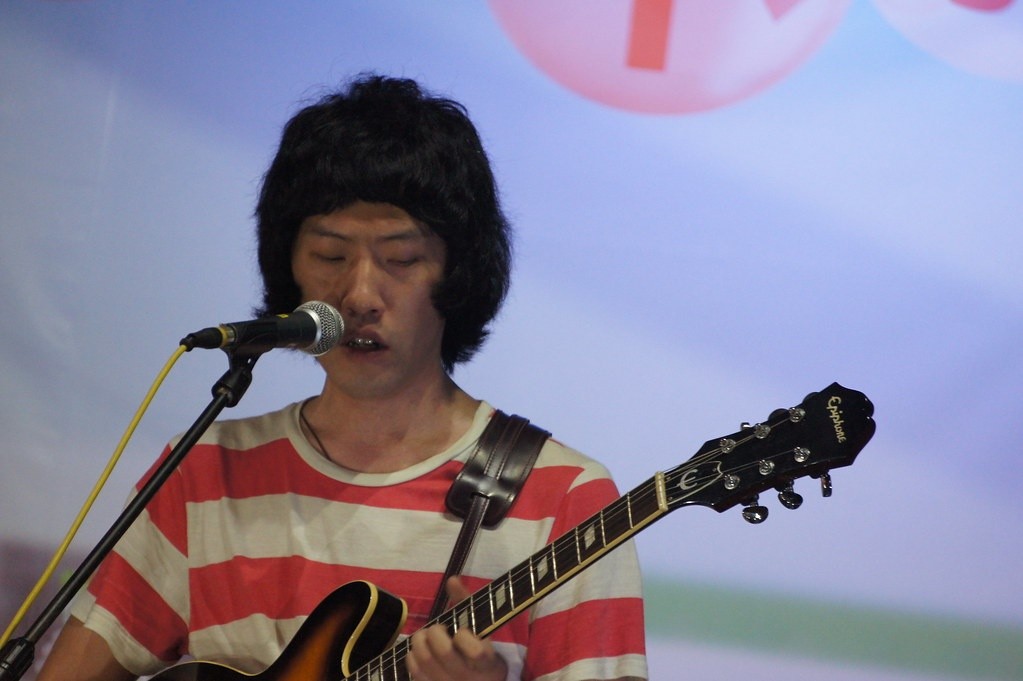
[181,299,345,356]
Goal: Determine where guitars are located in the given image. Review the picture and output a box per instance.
[148,381,880,681]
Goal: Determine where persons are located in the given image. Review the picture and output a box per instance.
[28,69,655,681]
